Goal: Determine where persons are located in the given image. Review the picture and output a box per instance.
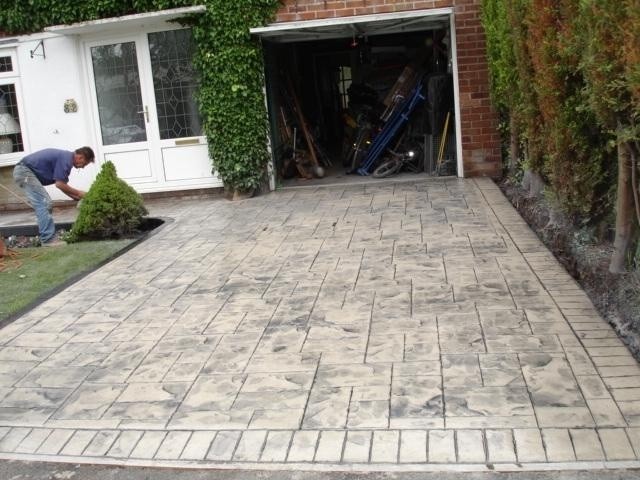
[13,146,95,248]
